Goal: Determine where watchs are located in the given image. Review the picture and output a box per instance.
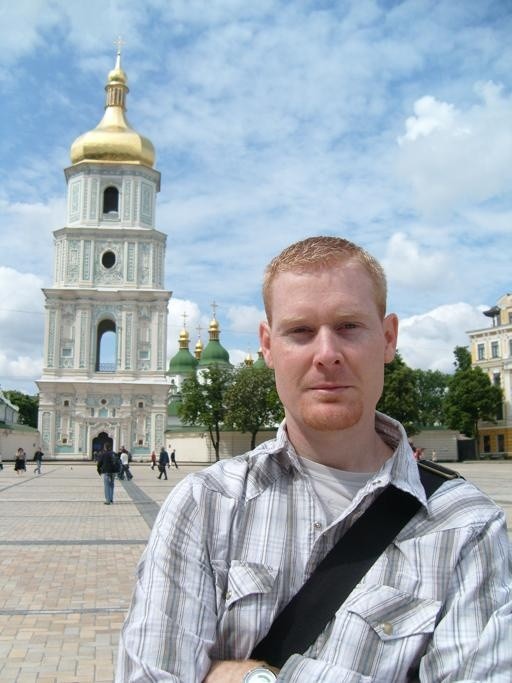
[243,664,280,683]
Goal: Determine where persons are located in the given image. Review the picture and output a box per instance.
[407,438,439,463]
[150,451,156,470]
[97,442,122,505]
[168,449,178,469]
[0,453,3,470]
[14,447,26,475]
[114,236,512,683]
[32,447,44,474]
[156,447,169,480]
[93,442,133,481]
